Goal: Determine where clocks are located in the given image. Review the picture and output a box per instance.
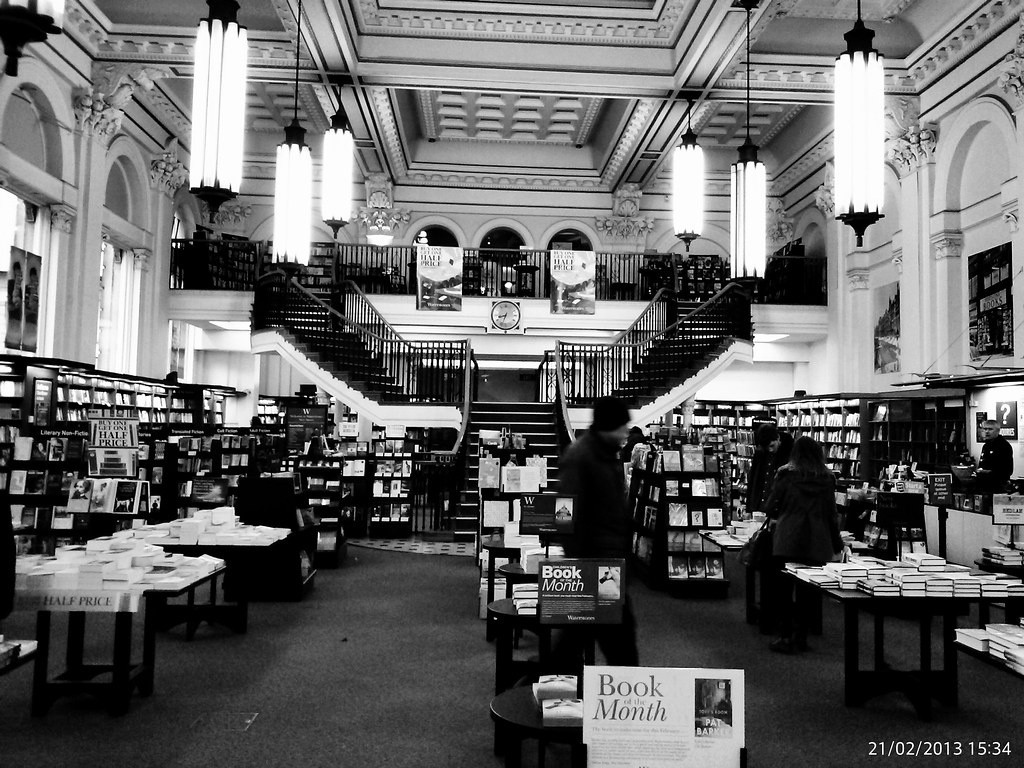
[487,300,524,335]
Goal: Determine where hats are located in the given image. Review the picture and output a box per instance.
[592,396,631,432]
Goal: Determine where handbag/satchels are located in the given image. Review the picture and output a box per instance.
[735,517,773,570]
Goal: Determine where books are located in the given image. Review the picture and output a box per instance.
[542,698,584,727]
[0,361,412,669]
[785,547,1024,677]
[478,429,565,615]
[632,400,860,581]
[537,675,578,702]
[533,683,542,705]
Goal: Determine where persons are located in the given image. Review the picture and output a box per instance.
[310,421,336,437]
[250,416,262,425]
[559,396,640,668]
[764,436,845,653]
[974,419,1014,516]
[744,424,799,633]
[620,425,657,463]
[774,429,795,478]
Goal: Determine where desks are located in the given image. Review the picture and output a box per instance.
[482,542,570,647]
[780,568,1024,727]
[489,686,587,768]
[696,529,877,633]
[486,599,607,693]
[14,556,226,715]
[950,623,1024,684]
[498,561,599,598]
[85,525,291,646]
[975,558,1024,629]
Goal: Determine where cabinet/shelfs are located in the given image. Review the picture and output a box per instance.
[862,388,1024,564]
[631,395,862,598]
[0,356,412,580]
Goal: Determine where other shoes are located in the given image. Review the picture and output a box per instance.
[793,637,814,653]
[769,636,795,655]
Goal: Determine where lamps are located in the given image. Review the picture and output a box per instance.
[319,86,354,239]
[830,0,885,246]
[271,0,311,285]
[189,0,247,225]
[672,95,704,252]
[731,0,766,282]
